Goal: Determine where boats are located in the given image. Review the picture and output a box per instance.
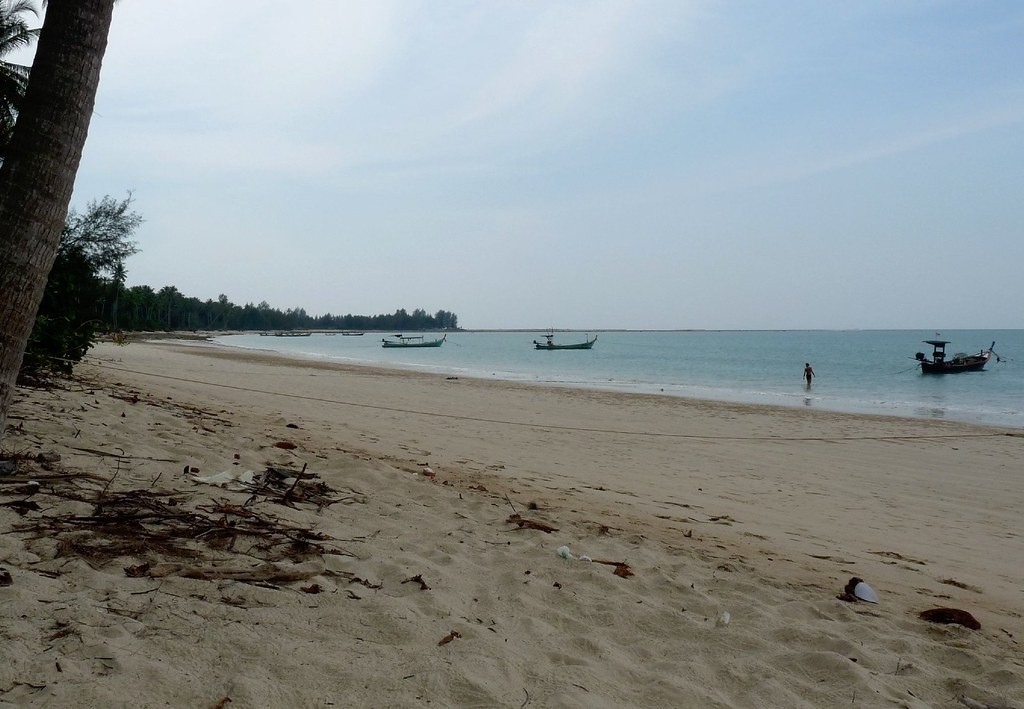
[342,332,364,336]
[380,333,448,348]
[258,332,280,336]
[275,331,313,336]
[533,334,598,351]
[915,340,996,376]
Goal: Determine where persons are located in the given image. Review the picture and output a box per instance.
[803,362,815,384]
